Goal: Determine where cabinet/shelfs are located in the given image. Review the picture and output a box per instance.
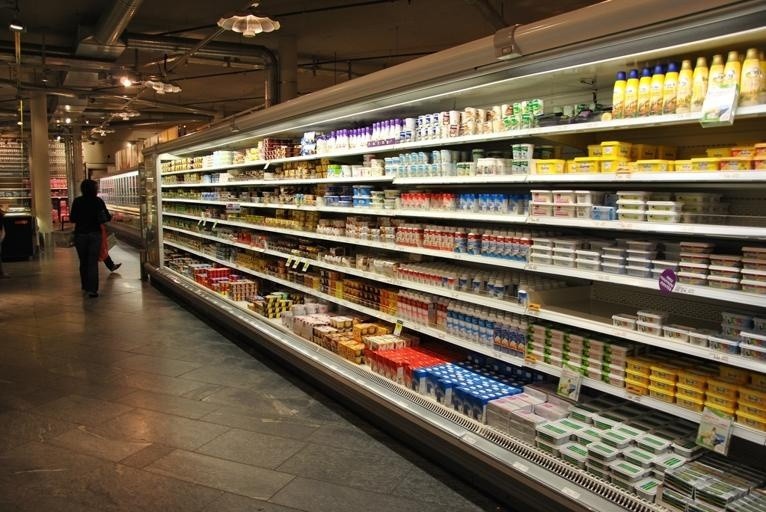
[141,0,766,512]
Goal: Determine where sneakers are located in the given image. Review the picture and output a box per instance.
[88,291,99,298]
[111,263,121,271]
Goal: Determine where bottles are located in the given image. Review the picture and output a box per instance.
[611,48,765,119]
[316,119,401,155]
[396,221,565,359]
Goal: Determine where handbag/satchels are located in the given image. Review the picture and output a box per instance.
[107,233,117,252]
[96,224,108,262]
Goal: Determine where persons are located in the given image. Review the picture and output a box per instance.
[0,208,11,279]
[92,180,121,272]
[69,179,112,298]
